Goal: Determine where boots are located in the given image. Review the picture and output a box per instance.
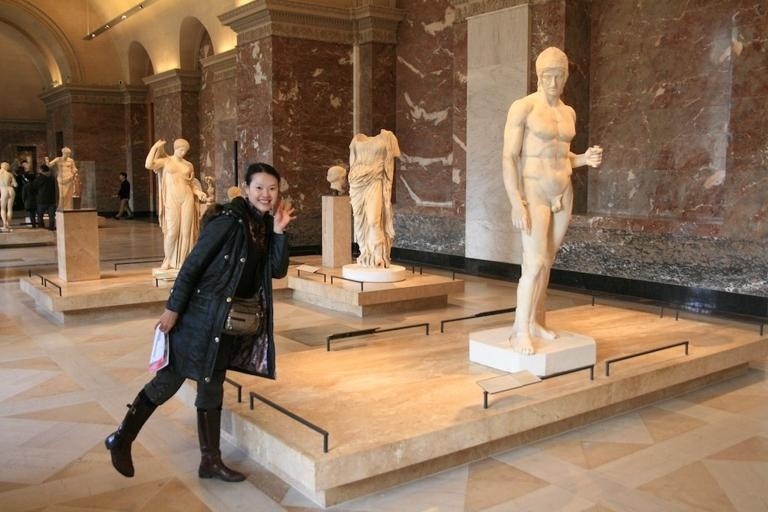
[104,388,158,477]
[196,407,245,482]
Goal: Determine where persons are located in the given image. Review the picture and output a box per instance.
[144,137,208,272]
[326,166,347,196]
[347,128,401,268]
[21,173,38,228]
[45,147,79,209]
[500,45,603,357]
[31,163,57,231]
[104,161,298,483]
[113,171,134,220]
[0,162,19,229]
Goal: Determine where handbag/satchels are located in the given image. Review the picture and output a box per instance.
[221,294,265,336]
